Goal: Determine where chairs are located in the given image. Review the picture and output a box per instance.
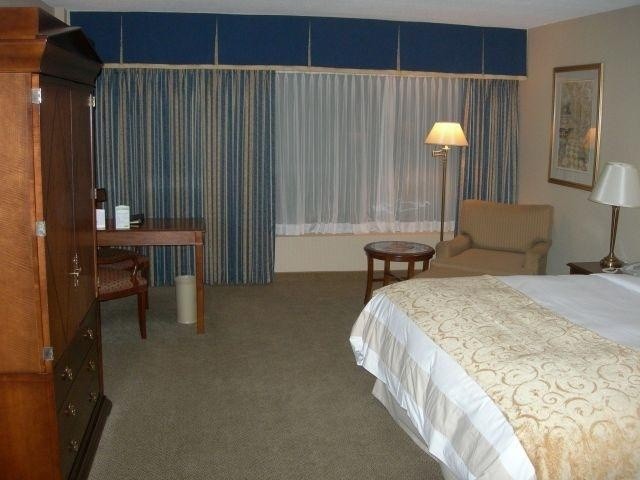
[96,188,151,307]
[428,201,554,277]
[96,267,149,340]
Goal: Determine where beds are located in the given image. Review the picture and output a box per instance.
[349,262,640,480]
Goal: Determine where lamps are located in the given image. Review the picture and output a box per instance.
[425,122,469,241]
[586,162,639,269]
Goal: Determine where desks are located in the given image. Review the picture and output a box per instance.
[98,221,206,335]
[364,242,435,303]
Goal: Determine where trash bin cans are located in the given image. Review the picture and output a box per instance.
[174,275,197,324]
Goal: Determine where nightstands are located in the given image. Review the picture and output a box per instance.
[566,261,632,272]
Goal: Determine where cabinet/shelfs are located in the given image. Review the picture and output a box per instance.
[2,8,111,478]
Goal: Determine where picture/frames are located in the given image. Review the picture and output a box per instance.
[548,63,604,191]
